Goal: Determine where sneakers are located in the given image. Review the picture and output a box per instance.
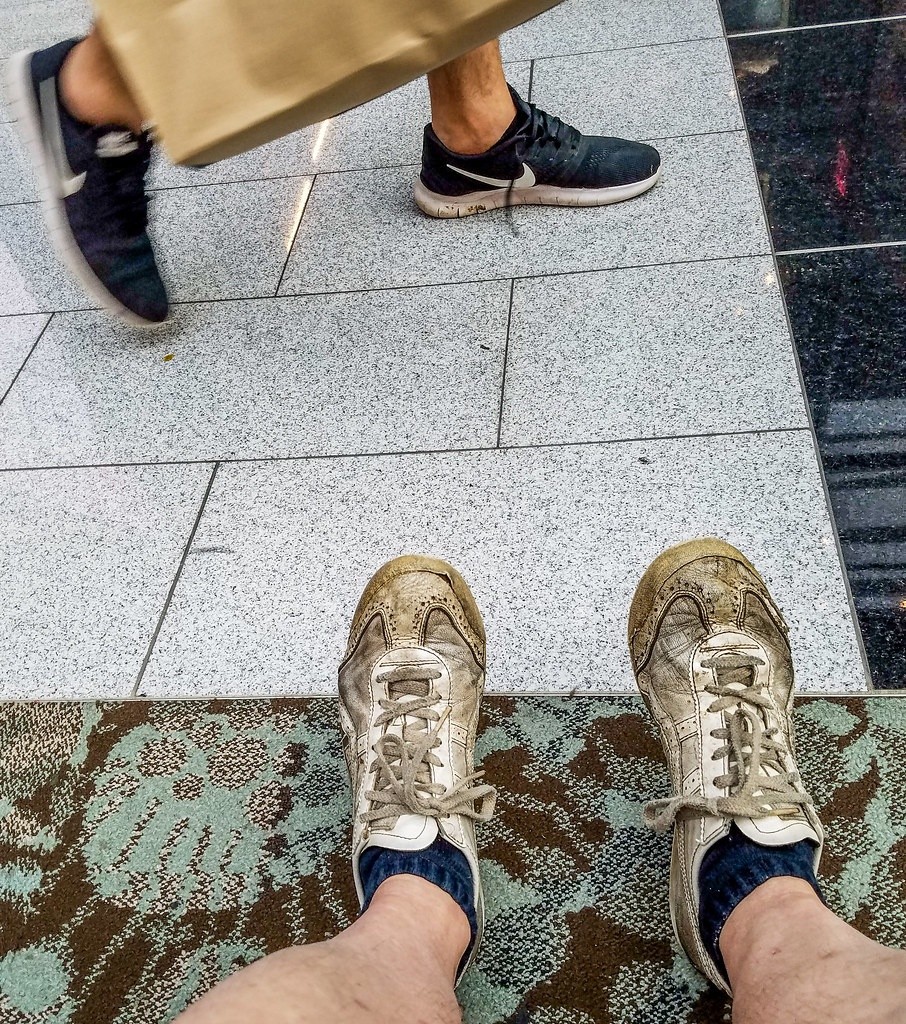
[333,553,489,982]
[630,538,823,990]
[415,82,661,216]
[11,36,174,324]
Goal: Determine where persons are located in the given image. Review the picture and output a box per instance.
[2,2,667,328]
[151,532,905,1022]
